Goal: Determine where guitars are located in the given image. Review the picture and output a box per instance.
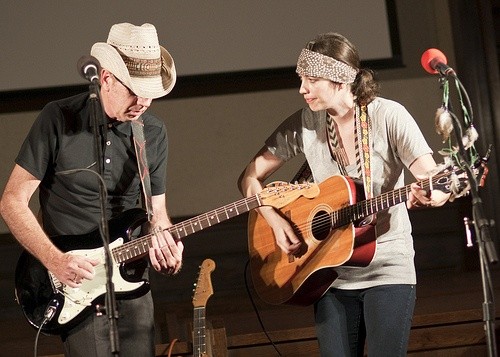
[248,157,486,308]
[191,258,216,357]
[12,181,320,337]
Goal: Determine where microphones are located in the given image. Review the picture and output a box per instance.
[77,55,100,85]
[421,48,457,79]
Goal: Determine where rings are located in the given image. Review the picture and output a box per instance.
[73,274,77,282]
[157,258,164,263]
[412,199,419,206]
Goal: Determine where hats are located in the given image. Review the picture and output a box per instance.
[90,22,176,99]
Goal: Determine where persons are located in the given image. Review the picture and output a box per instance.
[236,31,453,357]
[0,22,184,357]
